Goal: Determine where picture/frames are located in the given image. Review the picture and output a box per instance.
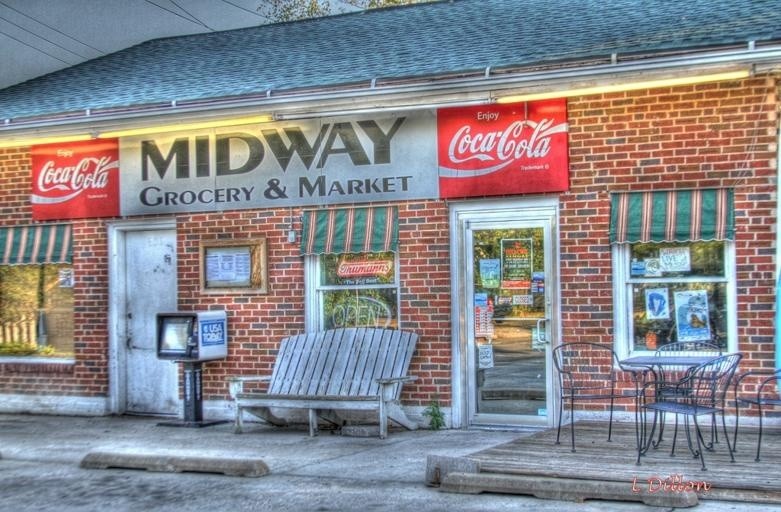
[198,237,270,296]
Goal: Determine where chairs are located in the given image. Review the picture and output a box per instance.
[551,341,781,473]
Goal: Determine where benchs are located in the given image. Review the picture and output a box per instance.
[225,326,422,439]
